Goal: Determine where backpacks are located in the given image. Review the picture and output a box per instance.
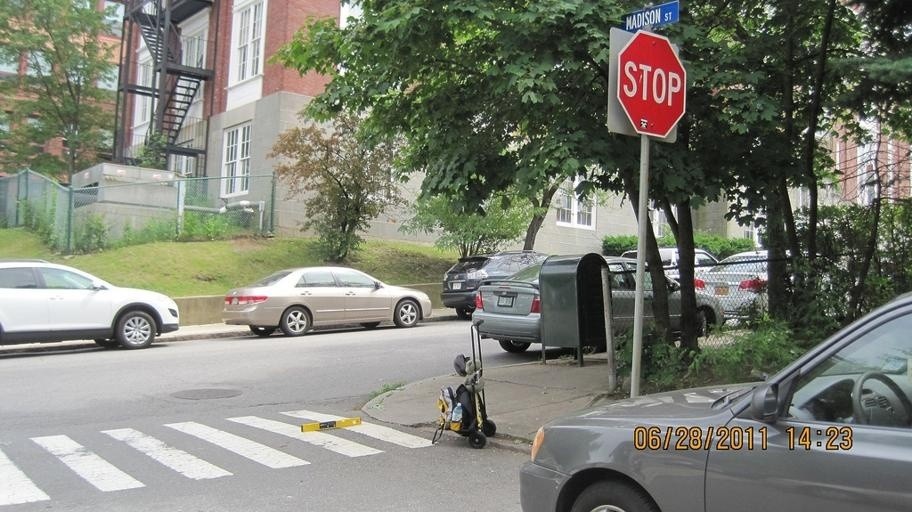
[437,384,488,437]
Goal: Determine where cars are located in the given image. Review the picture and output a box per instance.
[221,266,432,337]
[519,290,912,511]
[1,258,180,350]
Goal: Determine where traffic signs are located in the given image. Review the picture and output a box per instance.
[620,0,680,33]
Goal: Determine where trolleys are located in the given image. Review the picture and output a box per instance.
[432,319,498,450]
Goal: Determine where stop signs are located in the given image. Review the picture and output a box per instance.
[616,28,688,139]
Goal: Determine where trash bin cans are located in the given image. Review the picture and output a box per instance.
[538,252,613,367]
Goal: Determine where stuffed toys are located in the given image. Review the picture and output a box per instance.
[464,359,486,393]
